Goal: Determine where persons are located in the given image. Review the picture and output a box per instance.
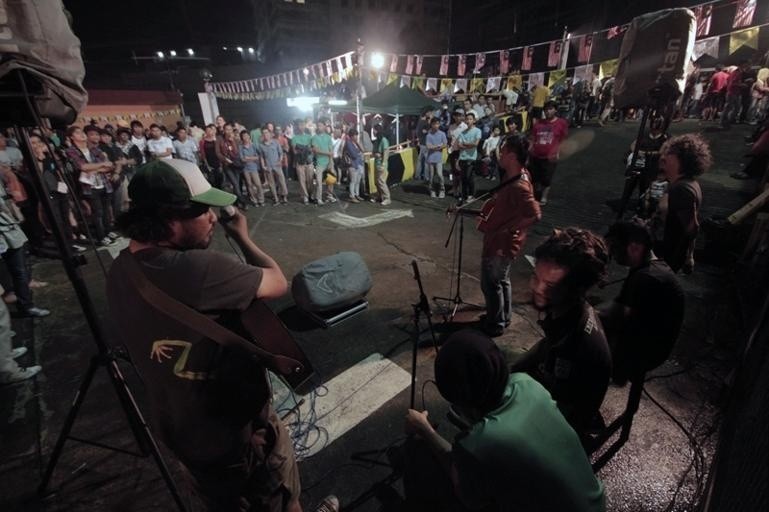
[595,210,686,392]
[1,118,92,385]
[702,65,769,131]
[64,116,242,246]
[238,117,393,206]
[106,154,301,509]
[573,77,636,128]
[401,329,607,511]
[467,131,543,336]
[656,77,681,133]
[416,81,550,199]
[642,134,711,280]
[507,226,615,437]
[388,116,417,146]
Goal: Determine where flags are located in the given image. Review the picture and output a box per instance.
[473,54,486,74]
[440,56,449,75]
[457,55,466,75]
[732,1,757,29]
[521,47,534,70]
[579,36,594,62]
[389,55,423,75]
[694,3,712,36]
[500,50,510,73]
[607,24,630,40]
[548,41,563,67]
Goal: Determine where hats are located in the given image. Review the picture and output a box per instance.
[128,158,238,207]
[434,334,509,408]
[454,108,464,116]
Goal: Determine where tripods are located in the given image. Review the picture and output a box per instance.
[433,215,485,325]
[12,126,186,511]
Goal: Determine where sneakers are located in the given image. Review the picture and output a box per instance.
[311,495,341,511]
[4,279,51,381]
[429,186,478,204]
[235,196,393,211]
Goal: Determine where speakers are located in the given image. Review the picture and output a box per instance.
[620,7,695,107]
[1,1,78,127]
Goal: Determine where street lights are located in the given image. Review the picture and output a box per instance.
[201,69,218,124]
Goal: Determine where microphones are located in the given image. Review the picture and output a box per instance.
[220,205,235,220]
